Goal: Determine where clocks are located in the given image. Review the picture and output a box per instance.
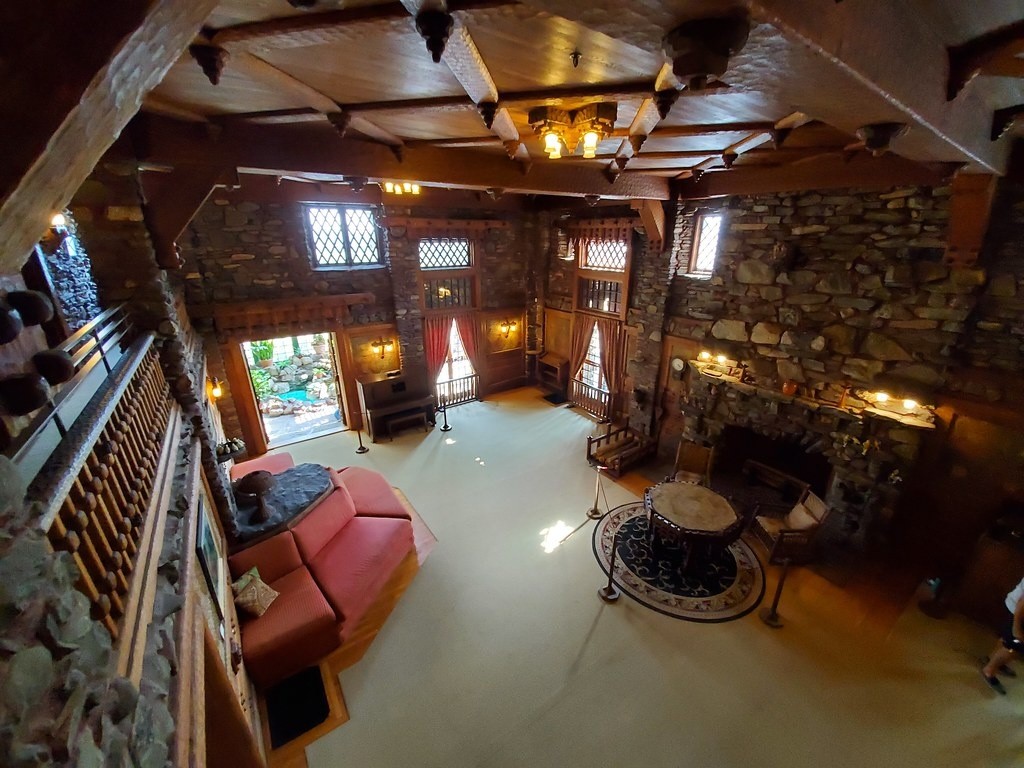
[671,355,686,380]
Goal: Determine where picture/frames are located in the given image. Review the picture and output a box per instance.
[196,493,223,621]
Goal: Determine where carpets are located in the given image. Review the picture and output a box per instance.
[592,500,766,623]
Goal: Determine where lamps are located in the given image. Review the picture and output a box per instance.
[371,335,393,359]
[501,317,517,339]
[211,376,222,397]
[528,101,618,159]
[48,213,67,235]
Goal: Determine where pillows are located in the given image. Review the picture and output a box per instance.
[232,564,261,595]
[234,577,280,617]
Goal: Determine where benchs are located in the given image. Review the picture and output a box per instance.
[742,460,815,503]
[384,409,428,442]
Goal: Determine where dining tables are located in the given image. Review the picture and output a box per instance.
[643,473,745,570]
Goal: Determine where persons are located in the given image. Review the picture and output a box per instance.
[979,576,1024,696]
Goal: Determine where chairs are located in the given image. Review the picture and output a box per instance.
[749,487,831,566]
[670,438,715,489]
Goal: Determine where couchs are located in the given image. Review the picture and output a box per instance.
[231,451,420,694]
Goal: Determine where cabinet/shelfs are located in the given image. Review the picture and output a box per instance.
[538,350,569,397]
[366,392,436,442]
[953,530,1024,627]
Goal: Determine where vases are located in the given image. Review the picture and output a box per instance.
[782,380,798,394]
